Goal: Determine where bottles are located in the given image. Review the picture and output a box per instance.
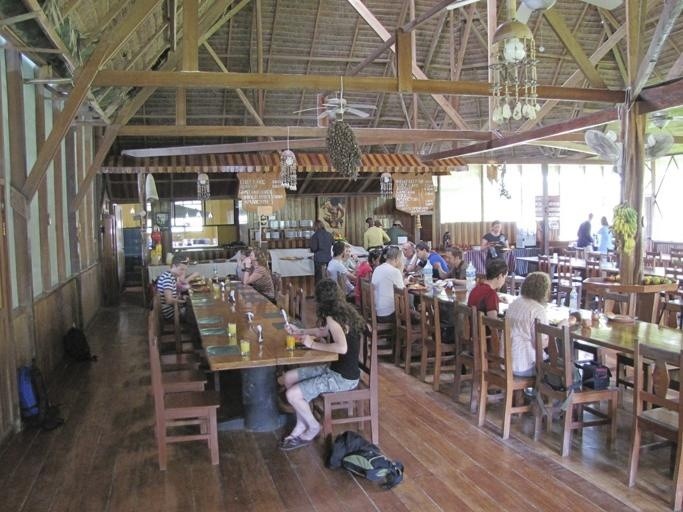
[591,295,599,321]
[465,262,476,291]
[422,260,432,287]
[568,287,577,312]
[245,309,265,344]
[220,272,238,313]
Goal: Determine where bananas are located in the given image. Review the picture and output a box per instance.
[612,207,638,255]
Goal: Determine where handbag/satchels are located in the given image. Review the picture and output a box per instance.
[432,325,454,343]
[544,358,582,391]
[582,361,612,390]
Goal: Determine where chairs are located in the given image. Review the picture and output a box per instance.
[226,255,333,367]
[321,239,528,404]
[149,343,221,470]
[315,317,381,447]
[151,276,211,435]
[469,240,683,512]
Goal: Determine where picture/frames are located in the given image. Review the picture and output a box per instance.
[315,195,348,239]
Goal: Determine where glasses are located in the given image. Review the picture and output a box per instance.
[181,262,188,265]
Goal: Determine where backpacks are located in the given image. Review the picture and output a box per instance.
[18,365,64,431]
[342,449,404,488]
[61,326,98,371]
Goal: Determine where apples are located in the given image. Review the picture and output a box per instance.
[645,276,672,285]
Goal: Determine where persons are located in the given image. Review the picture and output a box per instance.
[507,271,580,423]
[276,279,363,452]
[311,207,510,335]
[154,247,276,325]
[575,213,594,248]
[465,256,508,363]
[597,216,615,252]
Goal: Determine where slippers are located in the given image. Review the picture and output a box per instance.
[278,436,313,451]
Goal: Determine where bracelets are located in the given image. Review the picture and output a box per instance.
[310,341,313,348]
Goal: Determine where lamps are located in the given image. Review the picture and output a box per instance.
[277,127,298,192]
[489,14,540,125]
[379,173,393,200]
[195,172,210,201]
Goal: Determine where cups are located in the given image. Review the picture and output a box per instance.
[286,335,295,351]
[213,284,219,295]
[239,339,250,358]
[226,319,236,337]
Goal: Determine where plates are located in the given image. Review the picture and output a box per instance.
[605,314,637,322]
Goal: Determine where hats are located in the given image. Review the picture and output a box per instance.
[393,220,403,227]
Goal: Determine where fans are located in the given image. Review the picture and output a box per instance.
[642,131,674,161]
[291,92,377,121]
[445,0,623,25]
[584,128,623,179]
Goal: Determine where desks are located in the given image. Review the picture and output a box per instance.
[187,276,336,434]
